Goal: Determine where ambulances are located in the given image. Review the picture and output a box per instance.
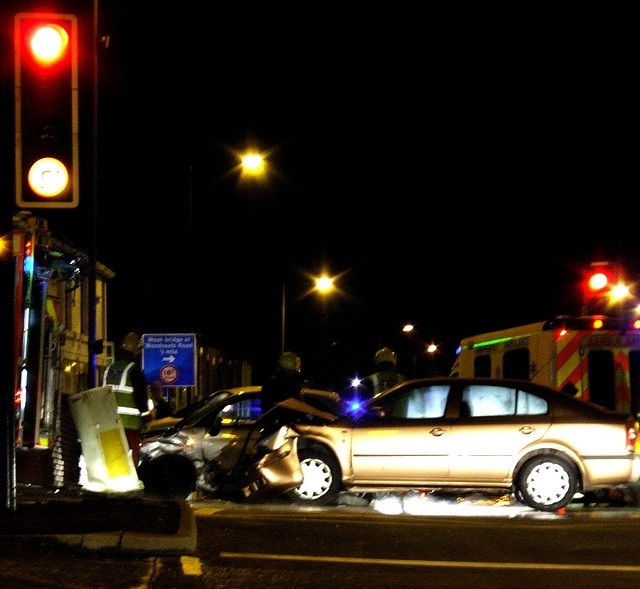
[448,315,639,419]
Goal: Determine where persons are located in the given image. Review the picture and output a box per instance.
[101,333,151,469]
[260,352,304,436]
[147,379,173,420]
[362,347,408,401]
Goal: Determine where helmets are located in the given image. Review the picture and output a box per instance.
[125,332,144,352]
[280,352,301,371]
[375,347,397,366]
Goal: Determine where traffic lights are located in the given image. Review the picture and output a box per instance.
[13,4,80,209]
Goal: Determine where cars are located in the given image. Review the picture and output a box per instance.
[244,378,639,513]
[139,386,354,501]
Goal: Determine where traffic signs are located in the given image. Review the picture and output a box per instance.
[141,333,198,388]
